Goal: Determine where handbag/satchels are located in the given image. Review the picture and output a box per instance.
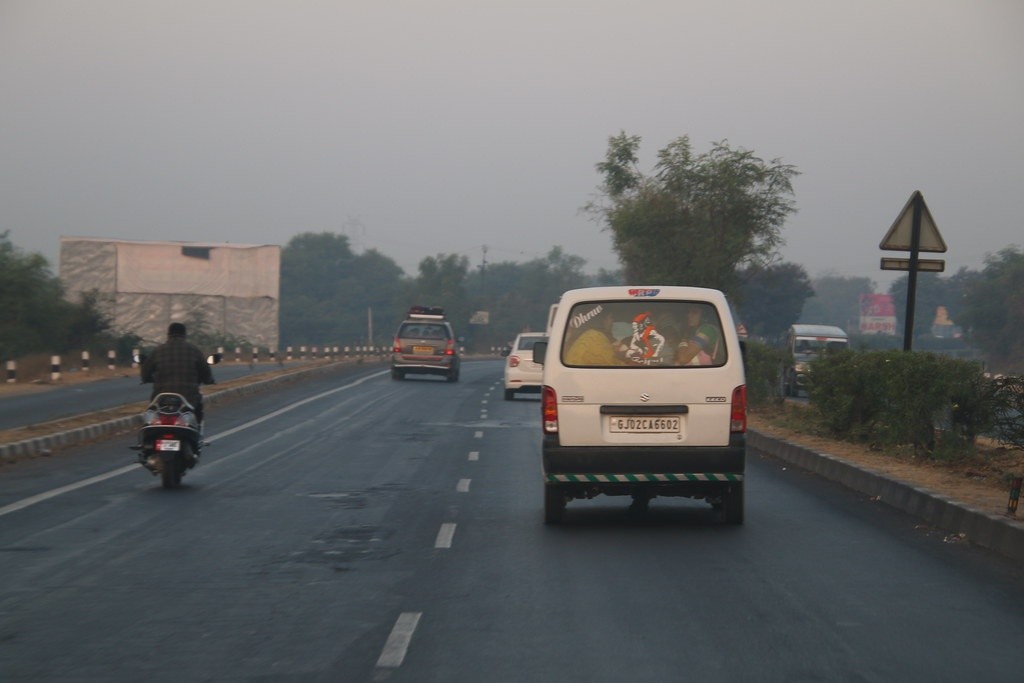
[676,325,719,366]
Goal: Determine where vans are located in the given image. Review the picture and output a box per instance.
[783,324,849,396]
[539,284,748,526]
[545,303,613,348]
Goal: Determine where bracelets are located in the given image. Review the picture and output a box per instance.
[677,342,689,347]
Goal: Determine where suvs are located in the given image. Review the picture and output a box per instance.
[390,307,461,380]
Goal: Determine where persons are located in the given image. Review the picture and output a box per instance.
[564,303,725,366]
[407,327,442,338]
[140,322,216,456]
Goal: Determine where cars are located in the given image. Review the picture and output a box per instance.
[500,331,549,399]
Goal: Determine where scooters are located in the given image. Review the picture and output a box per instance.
[128,354,224,490]
